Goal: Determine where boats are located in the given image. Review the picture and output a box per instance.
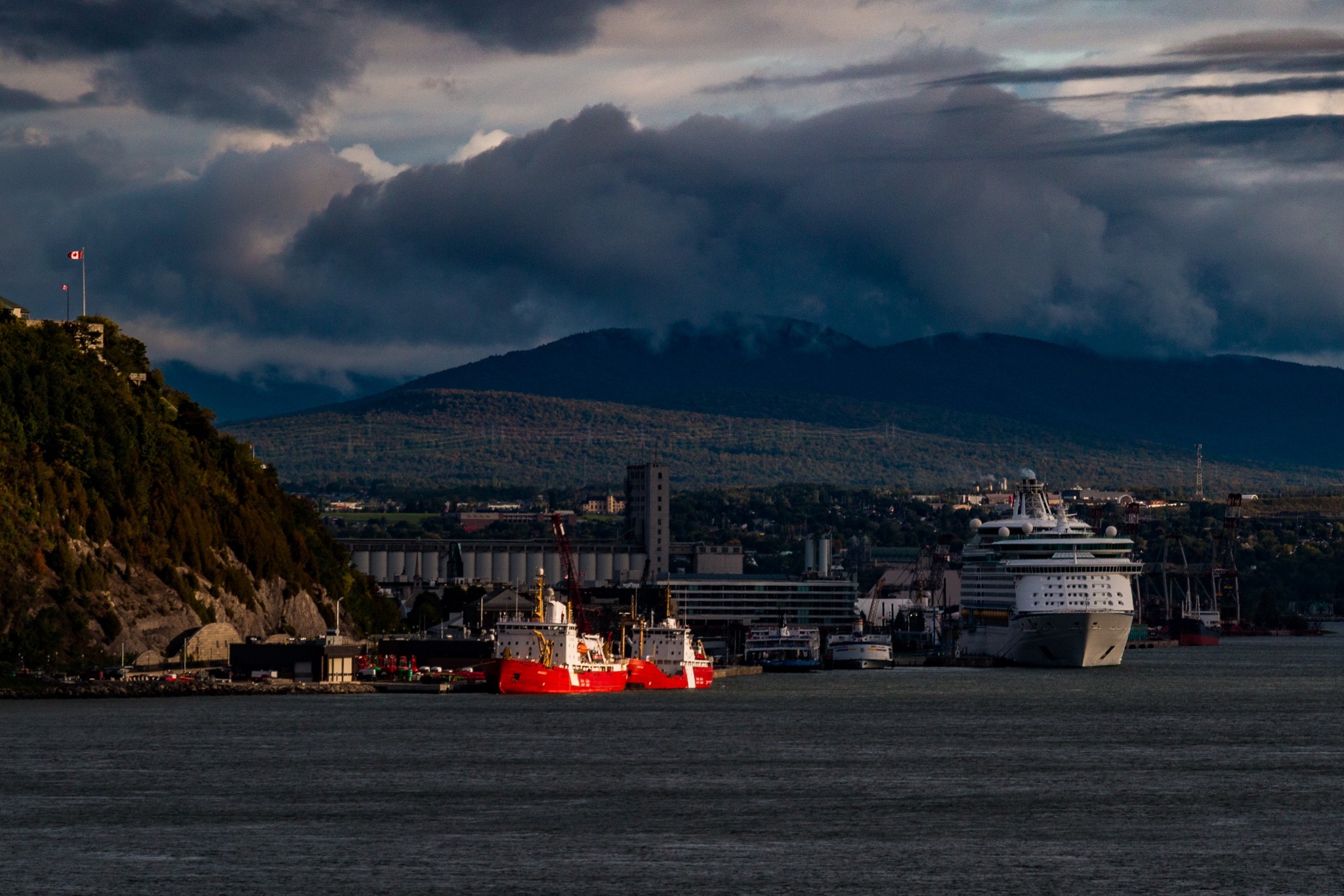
[822,615,894,668]
[490,567,630,694]
[623,568,716,690]
[1175,616,1221,646]
[743,605,820,670]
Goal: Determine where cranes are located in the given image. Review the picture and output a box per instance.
[551,510,589,635]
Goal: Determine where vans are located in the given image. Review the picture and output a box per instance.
[358,669,376,681]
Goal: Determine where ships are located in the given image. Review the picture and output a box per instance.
[930,464,1146,668]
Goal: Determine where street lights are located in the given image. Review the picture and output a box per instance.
[480,592,488,628]
[337,596,345,637]
